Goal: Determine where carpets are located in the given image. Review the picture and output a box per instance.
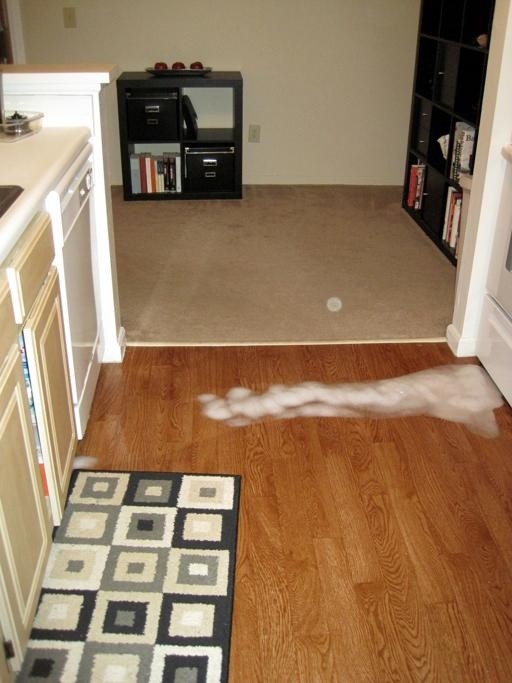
[109,184,456,347]
[18,468,241,682]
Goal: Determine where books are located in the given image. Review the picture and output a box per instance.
[405,161,427,211]
[128,150,181,193]
[440,121,476,247]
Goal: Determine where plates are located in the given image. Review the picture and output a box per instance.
[147,67,213,76]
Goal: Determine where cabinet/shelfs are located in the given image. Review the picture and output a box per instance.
[116,71,243,200]
[1,212,76,682]
[402,0,496,269]
